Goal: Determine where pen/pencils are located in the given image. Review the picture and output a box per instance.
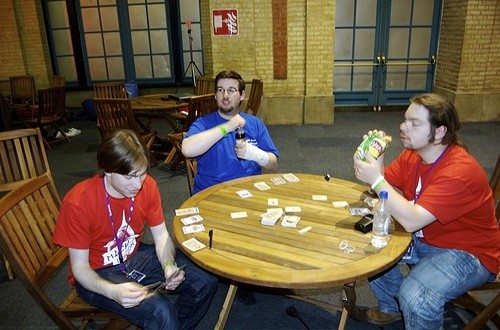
[209,230,214,248]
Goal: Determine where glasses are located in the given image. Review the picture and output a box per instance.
[216,86,239,96]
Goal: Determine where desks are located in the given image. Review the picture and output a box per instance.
[124,94,189,163]
[174,173,413,330]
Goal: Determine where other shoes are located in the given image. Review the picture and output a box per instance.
[236,286,256,305]
[365,307,403,325]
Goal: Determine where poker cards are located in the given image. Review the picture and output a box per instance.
[182,224,205,234]
[143,263,186,293]
[312,194,328,201]
[174,207,199,216]
[231,211,247,219]
[181,214,203,225]
[332,201,349,208]
[254,173,300,192]
[258,206,302,228]
[267,197,279,206]
[182,237,206,252]
[349,196,381,217]
[236,189,253,198]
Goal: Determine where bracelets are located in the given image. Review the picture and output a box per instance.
[217,124,227,137]
[162,260,178,271]
[371,174,384,190]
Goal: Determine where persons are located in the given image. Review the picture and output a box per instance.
[181,70,281,305]
[52,129,219,330]
[353,93,500,330]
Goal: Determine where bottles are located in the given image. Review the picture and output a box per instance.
[371,191,390,248]
[235,126,246,161]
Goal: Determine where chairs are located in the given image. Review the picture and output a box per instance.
[443,153,500,330]
[0,76,264,330]
